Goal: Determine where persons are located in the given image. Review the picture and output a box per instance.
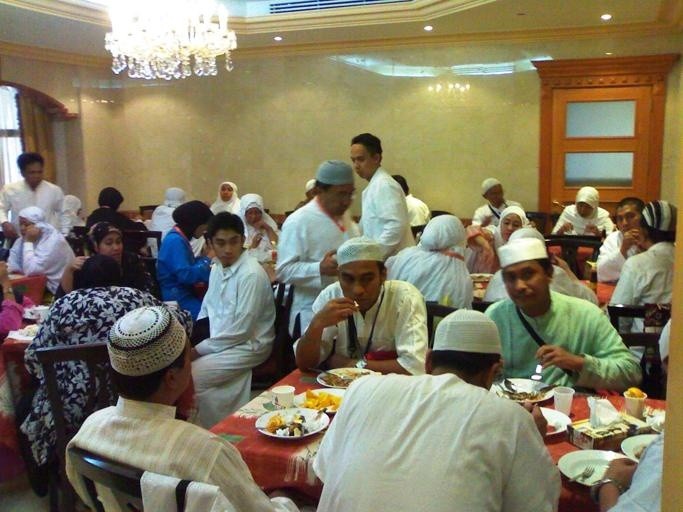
[66,306,299,511]
[590,317,671,512]
[276,136,677,393]
[313,309,562,512]
[0,153,279,510]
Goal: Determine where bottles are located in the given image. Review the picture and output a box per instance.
[590,262,597,295]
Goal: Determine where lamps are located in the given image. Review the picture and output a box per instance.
[104,1,237,80]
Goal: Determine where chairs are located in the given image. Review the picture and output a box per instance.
[544,234,602,281]
[73,225,94,257]
[36,343,118,511]
[122,229,161,259]
[608,304,672,399]
[252,281,295,390]
[135,253,163,303]
[473,301,493,312]
[427,301,459,349]
[526,211,547,232]
[68,446,218,512]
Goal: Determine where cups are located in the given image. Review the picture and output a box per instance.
[554,386,575,417]
[272,386,295,409]
[24,308,35,320]
[35,306,49,325]
[623,390,647,419]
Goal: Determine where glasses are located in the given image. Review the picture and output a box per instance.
[328,189,354,199]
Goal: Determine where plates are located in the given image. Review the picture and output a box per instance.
[489,377,555,403]
[650,422,664,434]
[563,231,596,237]
[622,434,663,462]
[559,448,638,487]
[295,388,344,414]
[541,406,572,439]
[470,273,496,283]
[255,407,330,440]
[315,366,380,390]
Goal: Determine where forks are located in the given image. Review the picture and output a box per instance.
[567,465,595,482]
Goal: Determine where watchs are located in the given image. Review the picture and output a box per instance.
[592,479,626,505]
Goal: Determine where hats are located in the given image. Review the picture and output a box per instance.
[337,237,383,265]
[482,178,501,195]
[496,239,548,268]
[106,307,186,377]
[305,179,316,192]
[433,309,500,353]
[316,161,352,185]
[641,201,676,232]
